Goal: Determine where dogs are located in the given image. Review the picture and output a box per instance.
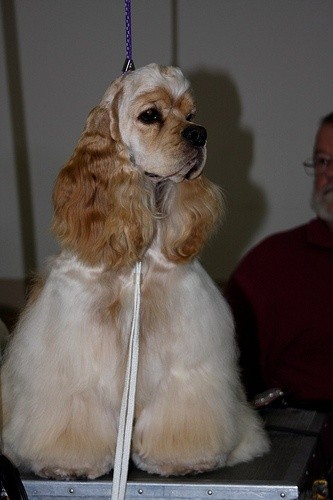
[1,62,272,481]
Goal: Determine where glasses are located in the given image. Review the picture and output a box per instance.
[303,156,333,178]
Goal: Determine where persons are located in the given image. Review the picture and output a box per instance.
[224,113,333,414]
